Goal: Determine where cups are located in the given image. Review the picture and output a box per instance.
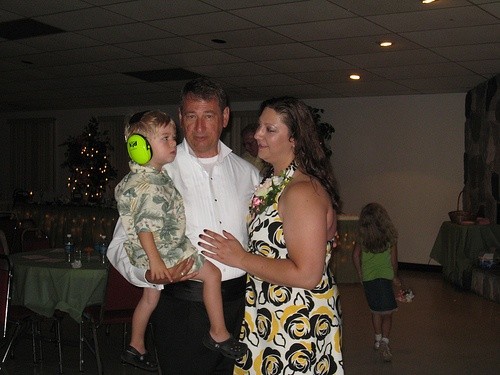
[84,245,93,256]
[73,249,82,261]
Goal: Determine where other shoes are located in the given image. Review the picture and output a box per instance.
[373,340,381,350]
[379,335,393,363]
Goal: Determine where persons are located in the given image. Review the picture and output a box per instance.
[353,203,400,363]
[242,125,265,172]
[106,78,262,375]
[198,99,345,375]
[114,109,249,369]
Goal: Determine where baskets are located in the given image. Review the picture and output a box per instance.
[448,191,476,222]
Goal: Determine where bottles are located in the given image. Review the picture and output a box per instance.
[64,234,74,263]
[99,235,108,264]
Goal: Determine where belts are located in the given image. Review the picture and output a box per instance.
[168,283,240,304]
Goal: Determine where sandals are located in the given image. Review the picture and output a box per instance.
[120,345,158,370]
[200,327,247,359]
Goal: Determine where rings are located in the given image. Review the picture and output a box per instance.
[181,272,185,276]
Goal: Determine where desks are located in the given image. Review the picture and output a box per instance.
[11,247,107,375]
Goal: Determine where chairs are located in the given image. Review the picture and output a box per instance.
[0,229,154,375]
